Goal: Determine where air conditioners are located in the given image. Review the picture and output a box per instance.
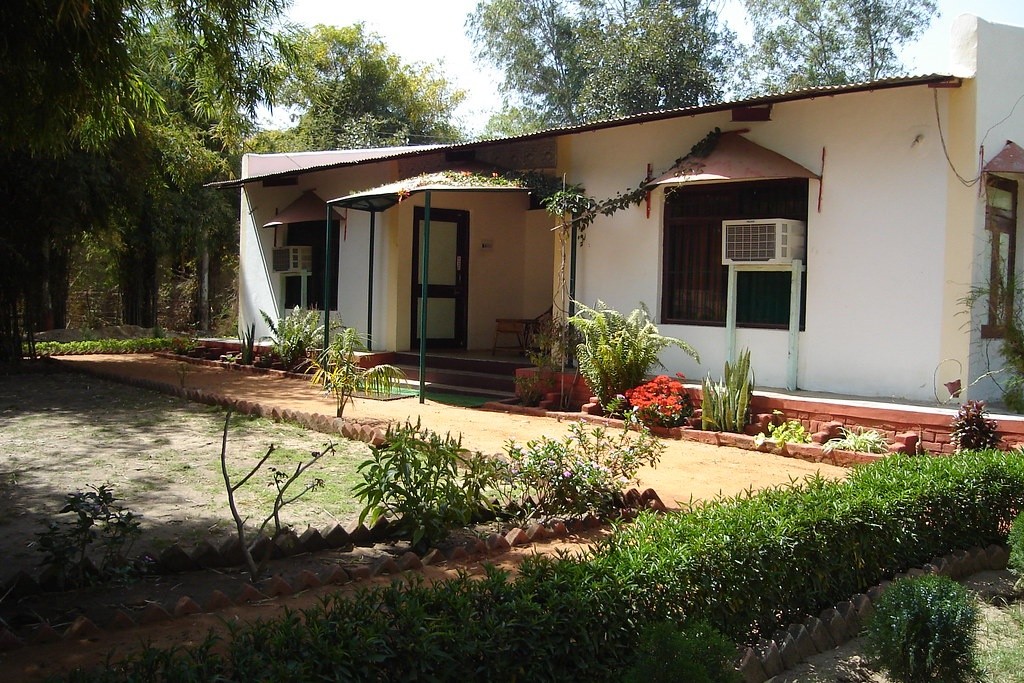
[722,219,806,266]
[272,245,312,274]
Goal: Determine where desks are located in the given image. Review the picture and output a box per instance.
[492,319,538,355]
